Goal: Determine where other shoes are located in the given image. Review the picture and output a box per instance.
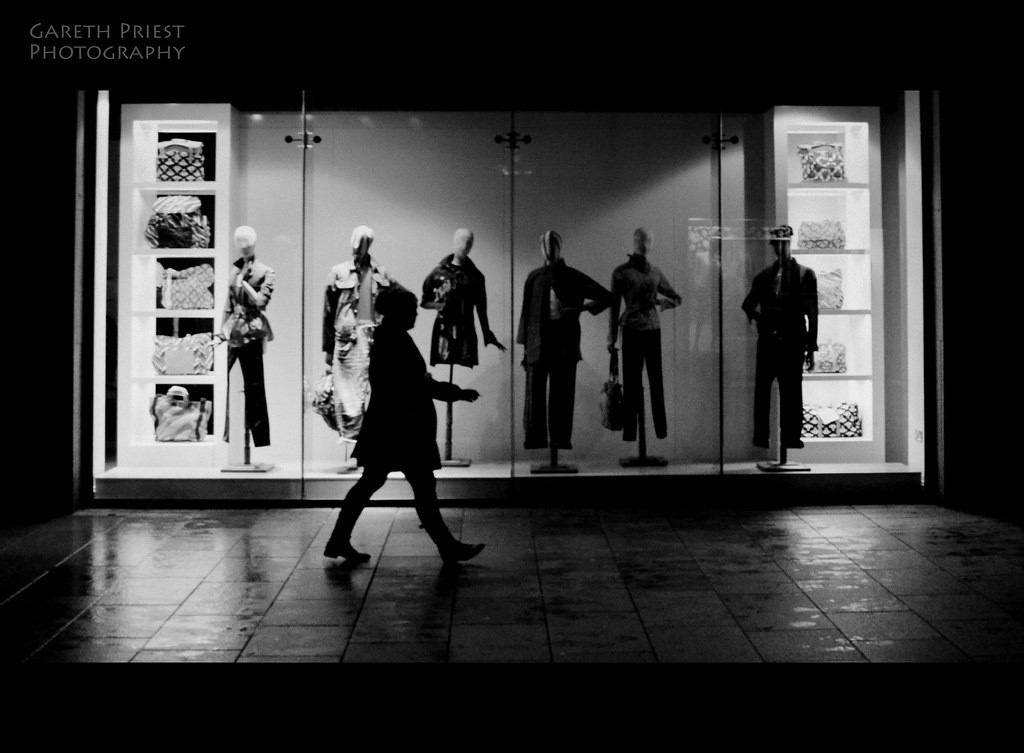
[442,538,485,562]
[325,537,370,564]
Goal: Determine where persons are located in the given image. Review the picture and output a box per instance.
[207,226,276,447]
[310,225,402,444]
[605,227,682,441]
[741,224,820,449]
[323,287,487,563]
[515,232,615,450]
[420,229,508,369]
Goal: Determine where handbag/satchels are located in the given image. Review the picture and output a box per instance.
[802,343,849,373]
[154,332,214,376]
[801,400,863,438]
[150,384,213,442]
[154,138,205,181]
[602,349,622,432]
[160,264,214,310]
[145,195,210,248]
[798,141,846,183]
[814,267,844,310]
[799,220,846,249]
